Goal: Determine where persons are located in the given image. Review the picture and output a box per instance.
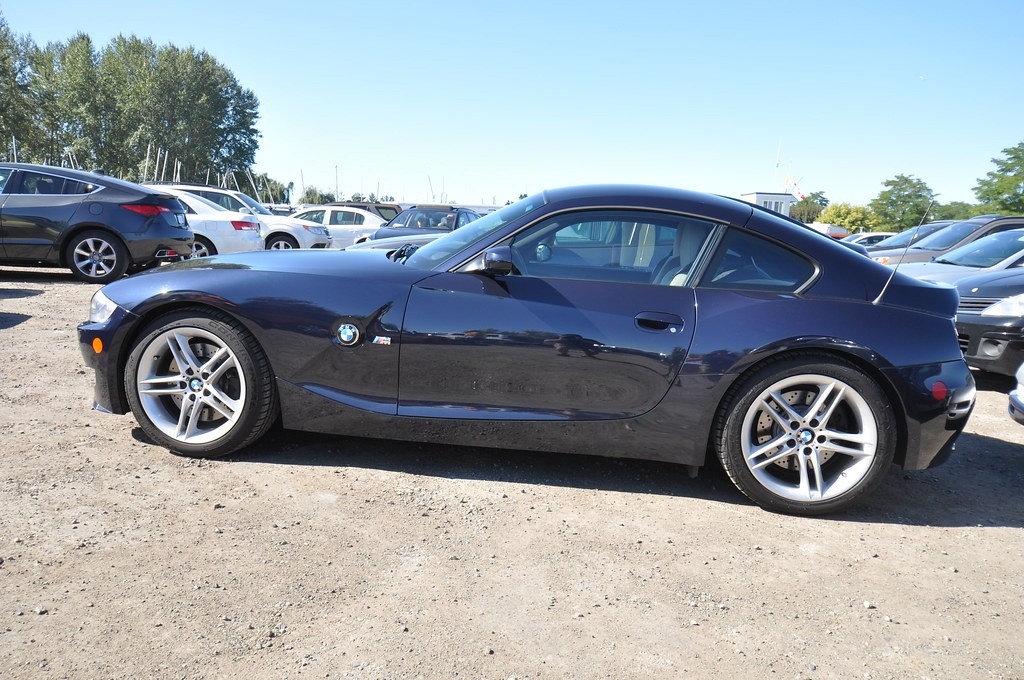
[438,215,455,229]
[36,180,52,194]
[546,332,602,360]
[701,350,734,389]
[665,347,685,379]
[417,216,427,228]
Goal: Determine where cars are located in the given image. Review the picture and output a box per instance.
[0,161,194,285]
[809,212,1023,425]
[365,204,484,242]
[345,220,674,270]
[141,186,264,263]
[261,199,403,251]
[72,182,977,518]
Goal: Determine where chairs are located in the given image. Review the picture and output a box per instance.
[659,221,709,290]
[647,217,687,284]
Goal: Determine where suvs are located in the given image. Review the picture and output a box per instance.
[141,180,333,252]
